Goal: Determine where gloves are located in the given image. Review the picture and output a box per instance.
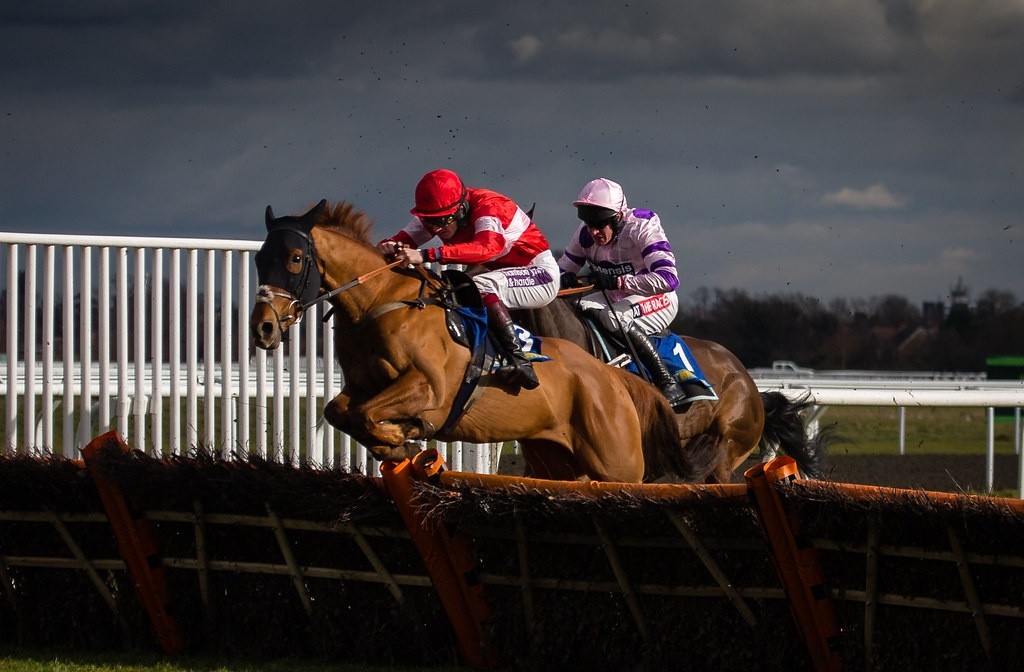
[561,272,578,289]
[588,271,619,290]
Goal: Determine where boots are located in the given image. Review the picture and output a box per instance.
[621,321,687,404]
[487,300,540,390]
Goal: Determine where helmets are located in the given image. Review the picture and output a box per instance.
[572,177,627,220]
[410,169,467,217]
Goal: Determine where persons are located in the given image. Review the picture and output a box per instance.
[376,170,561,391]
[557,177,686,405]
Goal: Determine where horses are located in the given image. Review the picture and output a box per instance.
[251,198,861,484]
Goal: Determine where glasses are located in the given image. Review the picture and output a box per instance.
[421,215,456,227]
[586,215,616,227]
